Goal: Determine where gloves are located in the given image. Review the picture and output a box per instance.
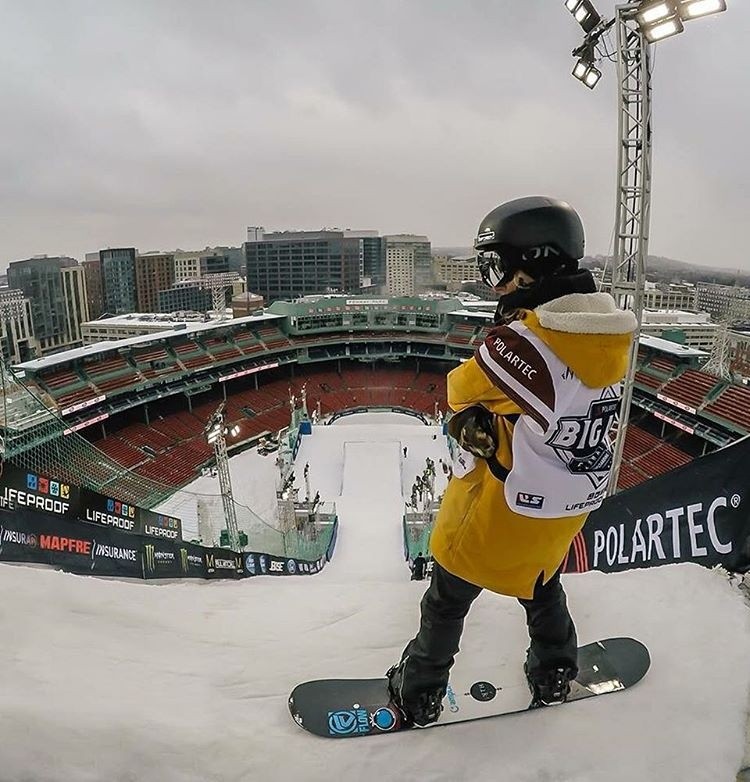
[448,406,496,459]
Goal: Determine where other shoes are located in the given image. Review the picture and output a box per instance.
[388,667,441,727]
[524,664,569,706]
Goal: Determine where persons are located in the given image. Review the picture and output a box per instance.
[387,196,640,724]
[416,457,436,501]
[304,464,309,479]
[404,446,407,457]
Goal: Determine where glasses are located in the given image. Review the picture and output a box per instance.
[478,250,506,289]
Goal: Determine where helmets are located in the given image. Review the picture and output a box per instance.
[473,197,588,262]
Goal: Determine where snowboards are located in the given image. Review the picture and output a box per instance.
[288,636,650,740]
